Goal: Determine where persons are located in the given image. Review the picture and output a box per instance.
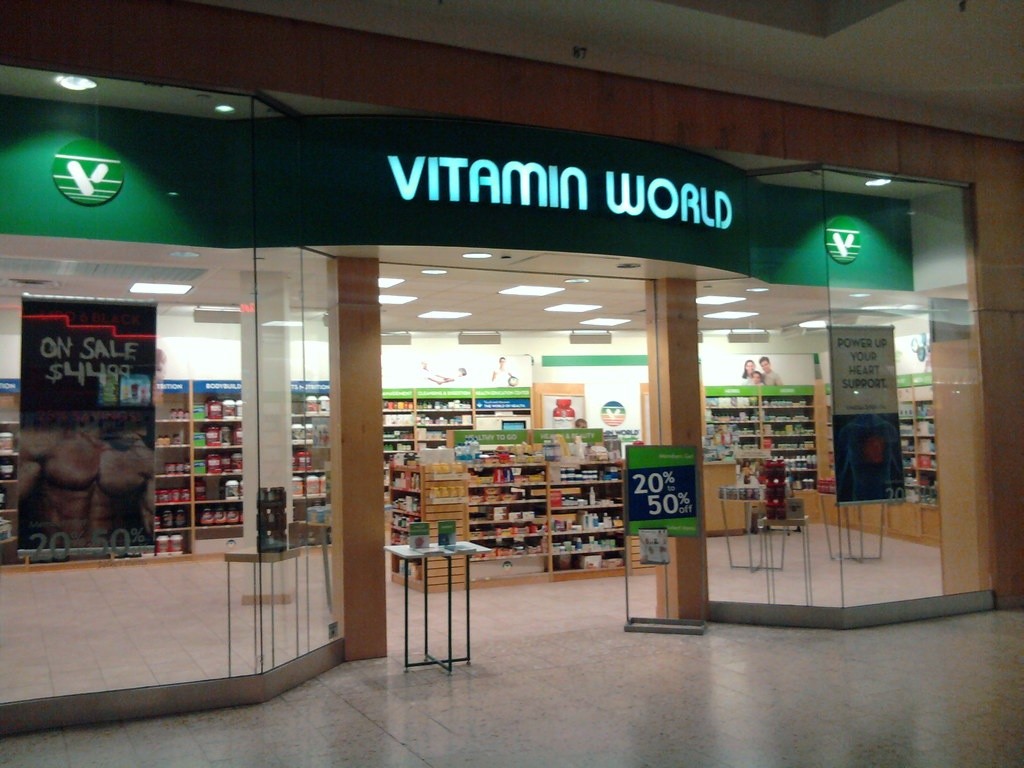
[492,357,512,387]
[15,426,154,548]
[741,356,782,386]
[421,360,469,388]
[575,418,587,428]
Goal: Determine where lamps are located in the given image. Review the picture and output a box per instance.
[569,330,612,344]
[381,331,412,345]
[458,331,502,344]
[727,329,769,343]
[781,322,806,340]
[194,304,240,323]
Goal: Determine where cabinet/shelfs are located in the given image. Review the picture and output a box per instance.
[704,372,942,548]
[388,457,657,594]
[0,377,533,583]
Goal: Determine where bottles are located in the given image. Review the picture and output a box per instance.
[578,511,599,529]
[464,438,469,445]
[472,438,479,457]
[590,487,596,505]
[553,399,576,429]
[455,443,472,463]
[784,474,794,497]
[769,455,817,471]
[468,438,472,445]
[603,513,612,529]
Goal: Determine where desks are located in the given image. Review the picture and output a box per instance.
[383,540,491,677]
[758,515,814,606]
[224,548,301,677]
[816,490,885,564]
[716,498,785,572]
